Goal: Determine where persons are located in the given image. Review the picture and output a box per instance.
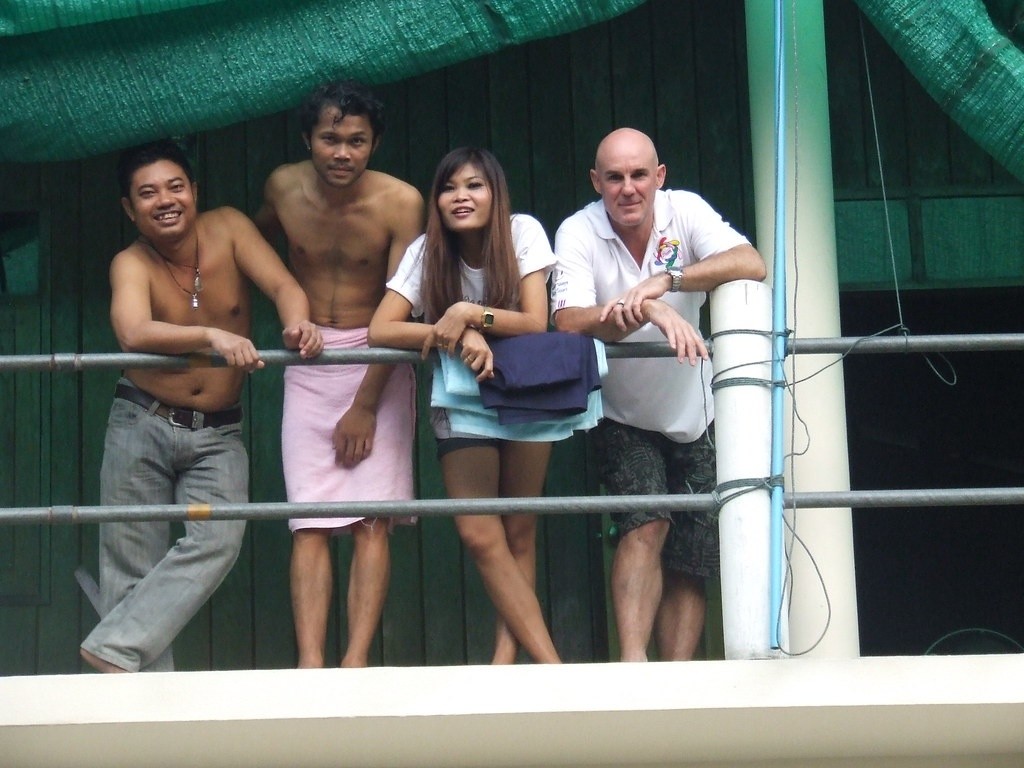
[366,146,561,665]
[548,127,768,662]
[77,134,325,671]
[250,81,427,669]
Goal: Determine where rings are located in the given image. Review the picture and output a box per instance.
[436,343,443,347]
[442,345,448,349]
[616,303,624,307]
[467,357,473,363]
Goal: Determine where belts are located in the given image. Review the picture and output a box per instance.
[114,383,242,432]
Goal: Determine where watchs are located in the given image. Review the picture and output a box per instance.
[478,306,495,336]
[666,266,683,292]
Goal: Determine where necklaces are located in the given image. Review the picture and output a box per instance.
[137,225,208,312]
